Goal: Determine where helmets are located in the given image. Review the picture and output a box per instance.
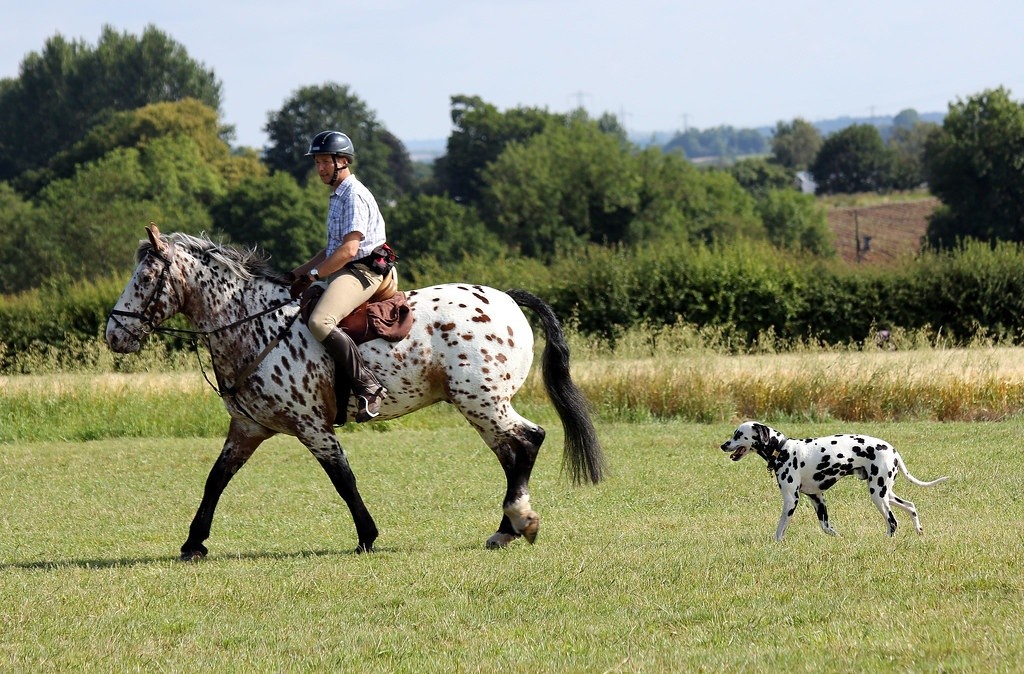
[304,130,354,164]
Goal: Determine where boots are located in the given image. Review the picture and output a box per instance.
[320,326,387,424]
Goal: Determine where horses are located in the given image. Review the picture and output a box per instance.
[104,222,607,562]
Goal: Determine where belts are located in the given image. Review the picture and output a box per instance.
[347,263,371,289]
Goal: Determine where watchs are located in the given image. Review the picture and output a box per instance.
[310,268,319,280]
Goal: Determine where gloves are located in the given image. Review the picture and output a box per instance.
[284,271,296,283]
[290,274,313,302]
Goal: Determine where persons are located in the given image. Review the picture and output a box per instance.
[286,130,387,421]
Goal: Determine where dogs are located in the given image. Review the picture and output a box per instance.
[720,421,952,543]
